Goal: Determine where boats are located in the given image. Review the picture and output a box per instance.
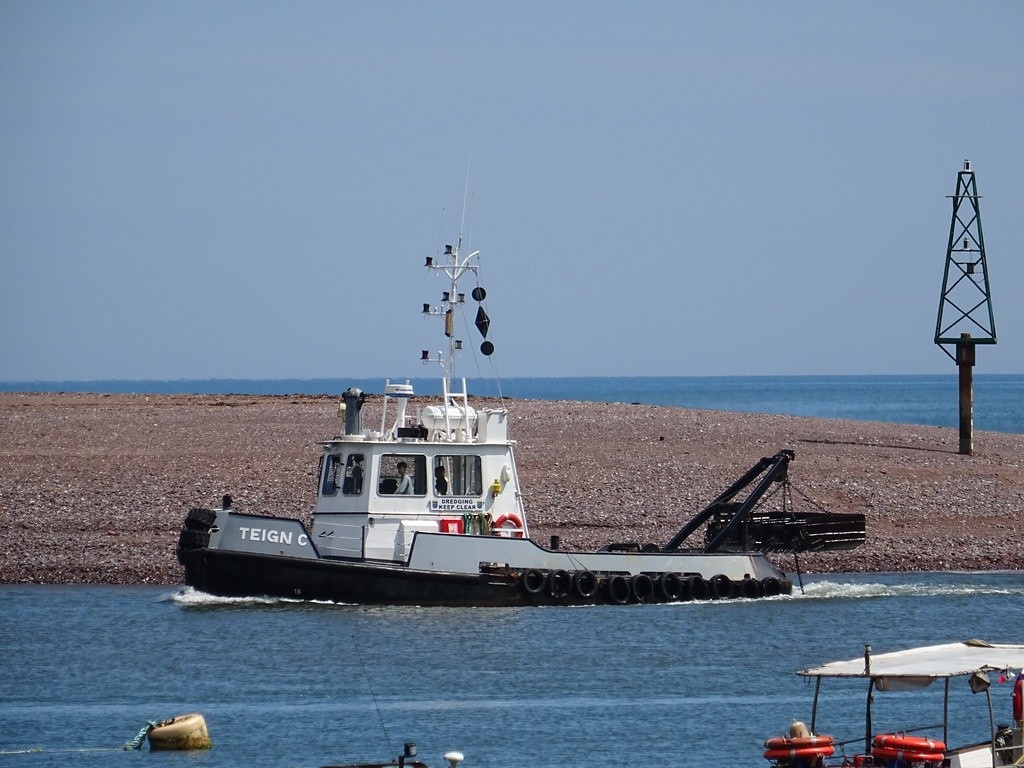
[758,639,1024,768]
[175,177,870,607]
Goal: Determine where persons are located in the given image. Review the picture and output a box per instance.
[346,466,362,494]
[393,461,413,495]
[435,466,449,495]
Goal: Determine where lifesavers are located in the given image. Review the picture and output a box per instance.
[658,572,683,600]
[521,566,546,595]
[872,747,945,761]
[738,578,764,599]
[629,574,654,603]
[546,568,572,599]
[1012,668,1024,726]
[763,746,835,758]
[874,734,945,752]
[762,735,834,749]
[758,576,781,598]
[687,576,711,603]
[494,513,523,538]
[605,575,631,604]
[710,574,731,598]
[573,570,599,597]
